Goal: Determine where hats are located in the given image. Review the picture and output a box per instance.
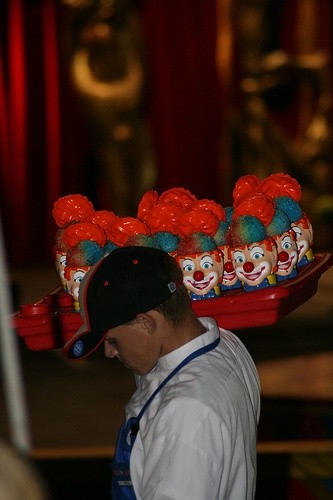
[61,245,184,361]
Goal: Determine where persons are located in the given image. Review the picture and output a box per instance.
[63,246,261,500]
